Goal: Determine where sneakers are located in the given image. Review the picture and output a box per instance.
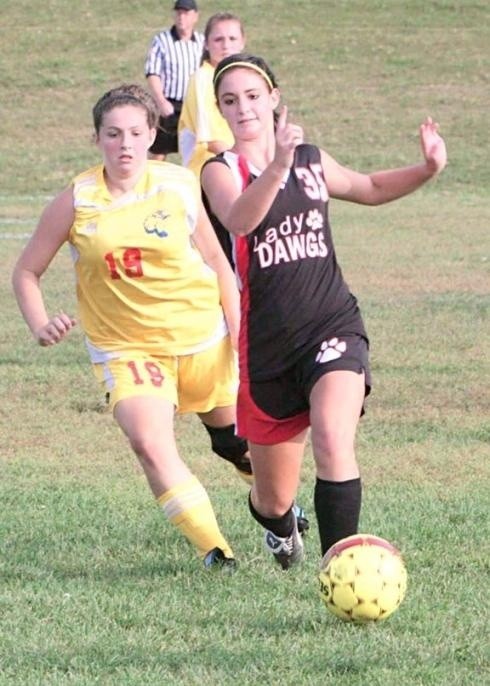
[264,505,309,570]
[203,547,237,571]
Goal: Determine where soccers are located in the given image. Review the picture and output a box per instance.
[319,534,407,624]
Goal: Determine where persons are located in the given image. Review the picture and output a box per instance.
[143,1,205,161]
[199,52,447,571]
[178,12,247,174]
[11,84,311,576]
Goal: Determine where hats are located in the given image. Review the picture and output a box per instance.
[174,0,197,11]
